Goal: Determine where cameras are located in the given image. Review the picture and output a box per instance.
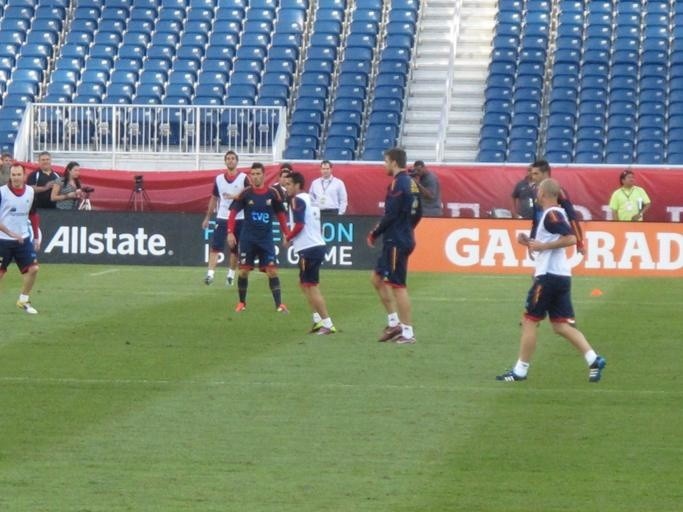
[83,187,95,193]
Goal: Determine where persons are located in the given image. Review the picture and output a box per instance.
[25,151,59,208]
[267,164,292,186]
[607,169,652,221]
[0,162,39,315]
[511,164,540,218]
[201,150,251,289]
[0,153,13,186]
[272,171,290,213]
[285,172,336,334]
[49,161,88,209]
[413,160,444,216]
[526,160,586,261]
[308,160,349,215]
[367,148,423,344]
[227,163,290,315]
[490,178,605,382]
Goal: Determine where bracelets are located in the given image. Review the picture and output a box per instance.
[638,212,643,217]
[575,237,583,247]
[64,193,68,200]
[418,184,422,188]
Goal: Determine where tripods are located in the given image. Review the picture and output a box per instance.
[127,189,152,212]
[79,192,91,211]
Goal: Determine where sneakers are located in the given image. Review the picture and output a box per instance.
[234,302,245,313]
[226,277,233,286]
[380,322,416,344]
[16,301,38,314]
[277,304,289,314]
[495,370,526,381]
[204,276,213,285]
[588,355,607,382]
[309,323,336,336]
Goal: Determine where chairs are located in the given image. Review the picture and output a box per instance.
[0,0,683,166]
